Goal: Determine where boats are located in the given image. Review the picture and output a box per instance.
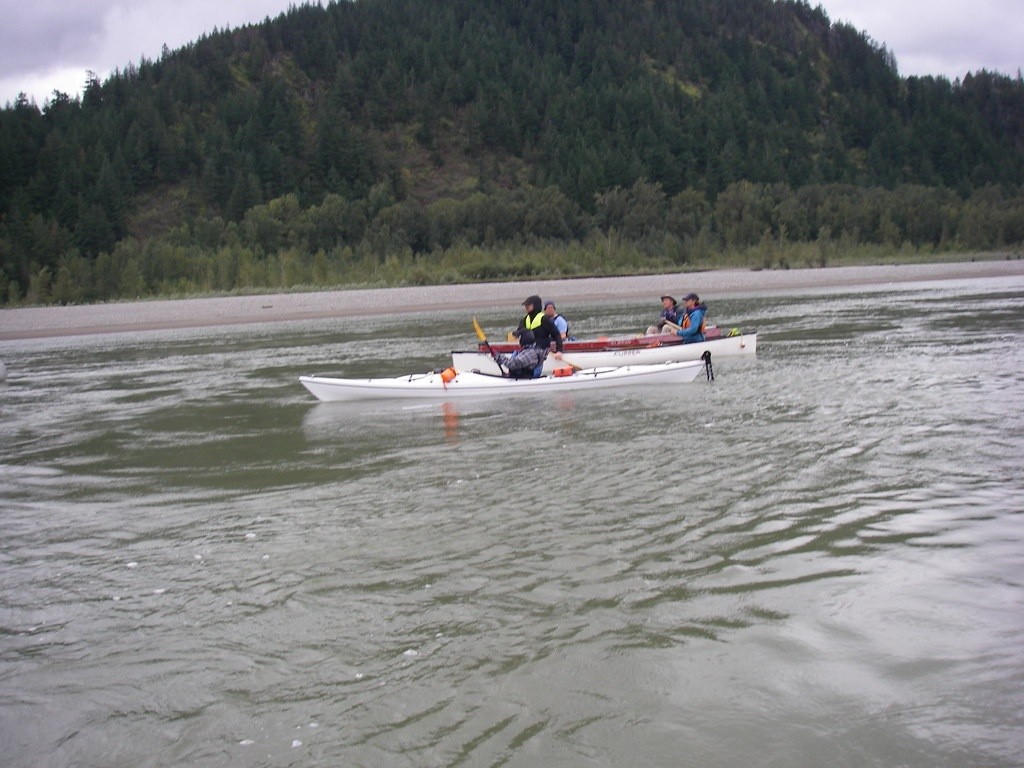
[452,324,760,376]
[296,346,712,405]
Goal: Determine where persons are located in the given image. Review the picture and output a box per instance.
[645,294,678,334]
[544,302,568,340]
[512,295,563,362]
[671,293,708,344]
[490,329,538,378]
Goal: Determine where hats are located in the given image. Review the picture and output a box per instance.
[522,296,541,315]
[512,327,534,346]
[661,294,677,305]
[682,294,699,302]
[544,301,556,311]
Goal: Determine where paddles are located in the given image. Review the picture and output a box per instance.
[473,318,505,375]
[507,331,518,344]
[560,358,580,371]
[664,317,682,330]
